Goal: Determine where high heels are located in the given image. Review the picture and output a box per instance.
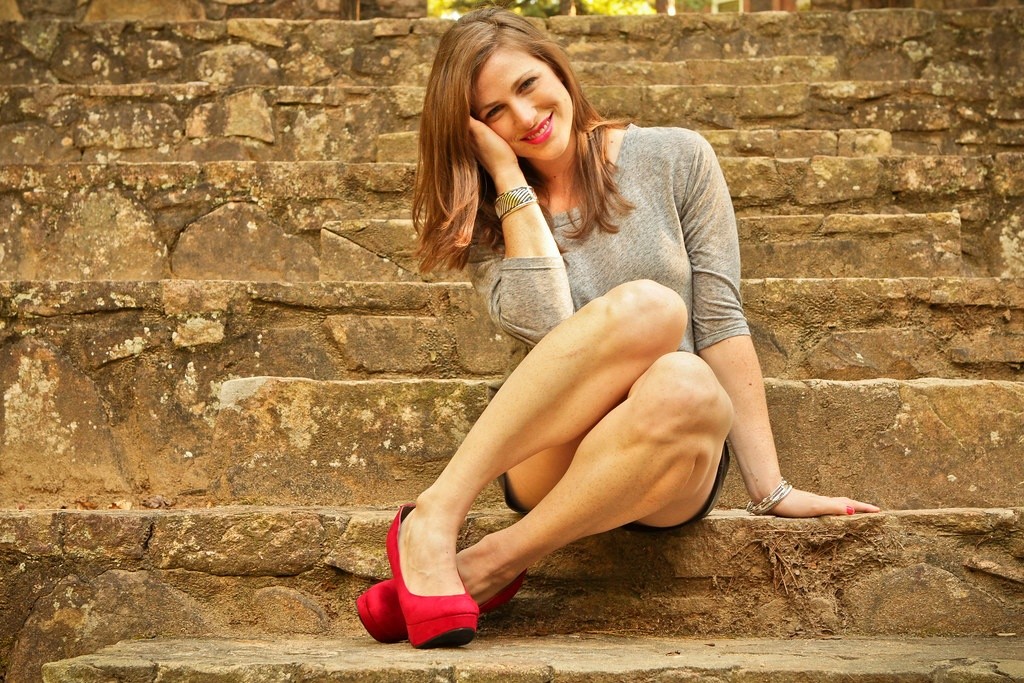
[388,501,478,648]
[355,548,526,644]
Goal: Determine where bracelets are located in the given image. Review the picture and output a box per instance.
[745,477,793,516]
[494,185,538,221]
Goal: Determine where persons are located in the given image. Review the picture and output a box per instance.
[355,9,881,646]
